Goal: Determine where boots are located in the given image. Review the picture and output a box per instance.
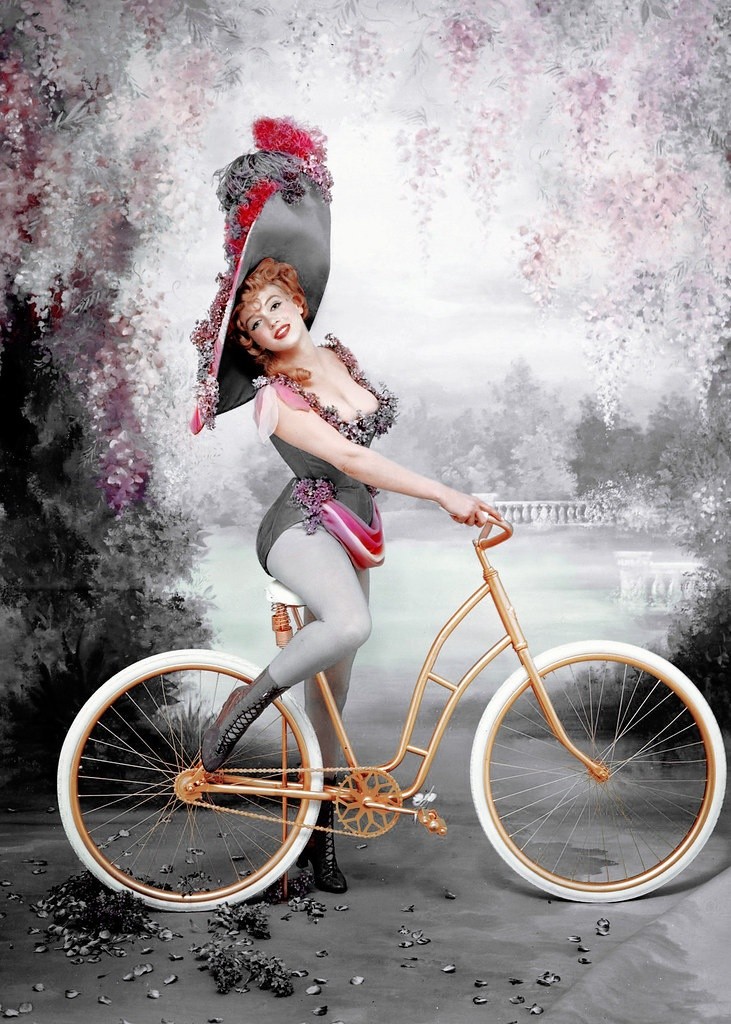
[296,775,348,894]
[200,665,292,773]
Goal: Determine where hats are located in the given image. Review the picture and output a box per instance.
[188,115,333,435]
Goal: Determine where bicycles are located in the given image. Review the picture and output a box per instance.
[55,506,730,915]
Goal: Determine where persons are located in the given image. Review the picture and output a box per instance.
[201,256,504,895]
